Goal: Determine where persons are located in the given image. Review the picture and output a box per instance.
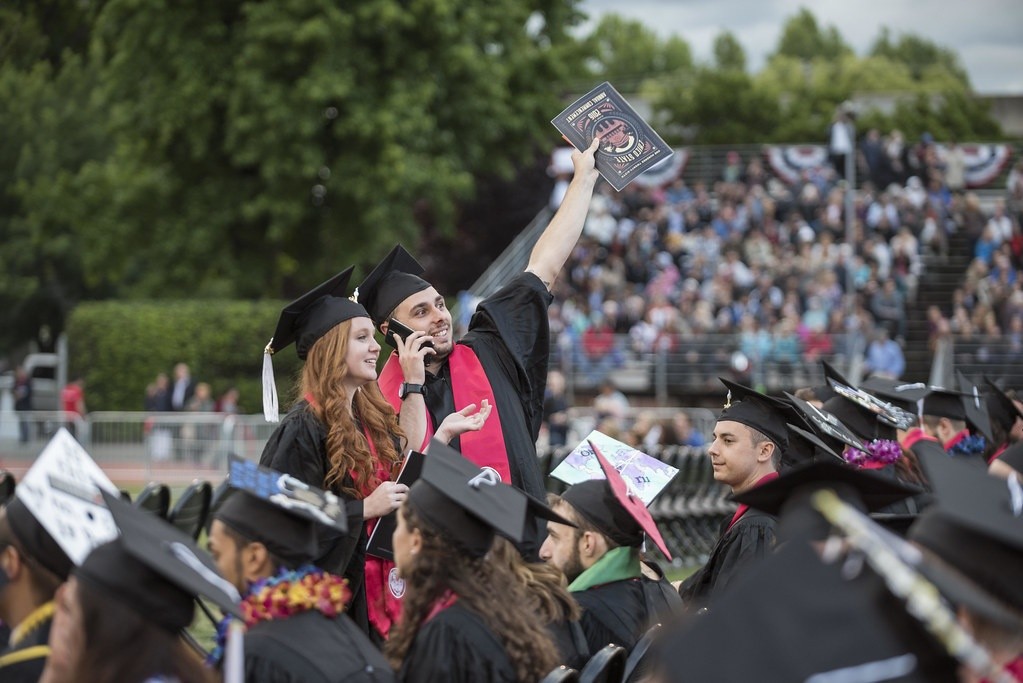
[0,358,1023,683]
[548,129,1023,445]
[354,137,599,503]
[263,266,493,642]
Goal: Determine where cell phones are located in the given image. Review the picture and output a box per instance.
[385,318,433,367]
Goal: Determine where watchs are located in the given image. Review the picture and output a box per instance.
[399,381,428,400]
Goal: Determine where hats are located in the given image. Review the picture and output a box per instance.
[354,244,432,334]
[513,482,578,560]
[563,438,675,565]
[411,434,529,556]
[662,358,1023,683]
[263,264,373,425]
[210,450,350,566]
[0,427,249,632]
